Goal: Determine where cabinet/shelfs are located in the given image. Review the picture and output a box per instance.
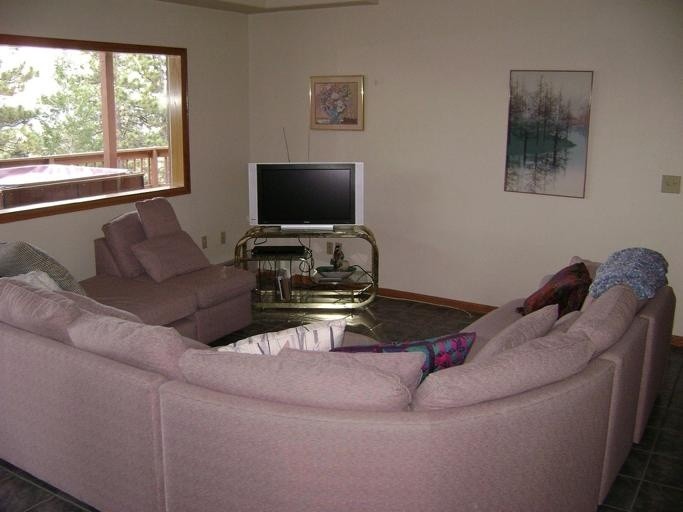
[232,226,381,311]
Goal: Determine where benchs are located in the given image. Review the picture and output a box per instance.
[78,263,256,345]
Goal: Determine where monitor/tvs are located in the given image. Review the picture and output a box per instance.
[247,161,366,232]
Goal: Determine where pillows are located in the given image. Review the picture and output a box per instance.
[94,196,211,284]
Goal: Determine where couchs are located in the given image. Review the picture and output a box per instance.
[0,276,677,512]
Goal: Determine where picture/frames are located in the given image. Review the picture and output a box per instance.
[501,68,595,201]
[306,73,367,134]
